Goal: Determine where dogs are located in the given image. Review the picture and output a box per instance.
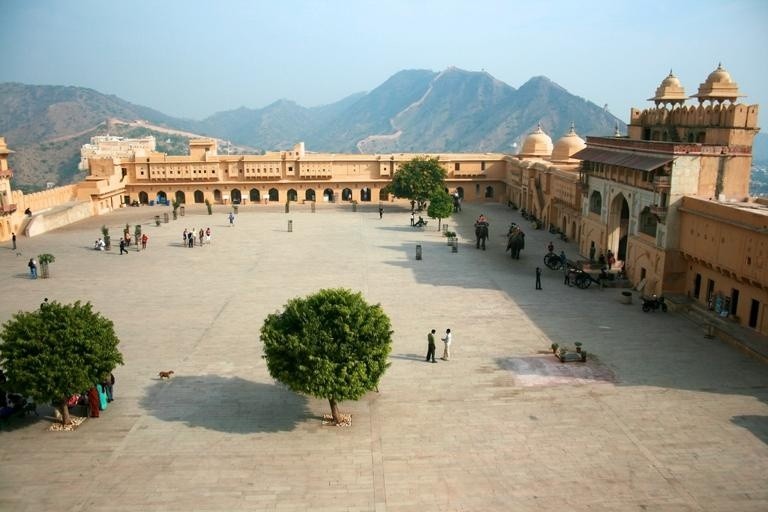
[158,370,174,379]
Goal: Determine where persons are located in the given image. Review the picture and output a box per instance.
[409,199,428,227]
[189,232,194,247]
[192,228,197,246]
[206,227,211,244]
[94,222,148,255]
[533,241,615,291]
[440,329,453,361]
[453,190,463,212]
[87,368,114,419]
[425,328,439,363]
[10,232,18,251]
[28,257,37,279]
[41,297,50,307]
[378,206,384,219]
[24,207,33,217]
[473,214,527,260]
[198,228,205,246]
[182,227,188,247]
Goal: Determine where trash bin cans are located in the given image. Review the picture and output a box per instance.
[452,237,458,252]
[181,206,185,217]
[312,203,316,212]
[288,220,292,232]
[154,215,160,222]
[416,245,422,260]
[621,291,633,305]
[234,206,240,214]
[164,212,168,223]
[442,224,447,235]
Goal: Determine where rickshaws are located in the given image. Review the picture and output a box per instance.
[570,267,608,293]
[544,251,579,272]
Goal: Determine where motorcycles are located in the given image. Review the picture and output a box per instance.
[639,292,668,313]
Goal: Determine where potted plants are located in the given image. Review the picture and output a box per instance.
[551,340,587,363]
[37,252,56,279]
[100,222,112,251]
[204,198,212,215]
[171,191,185,220]
[444,231,458,246]
